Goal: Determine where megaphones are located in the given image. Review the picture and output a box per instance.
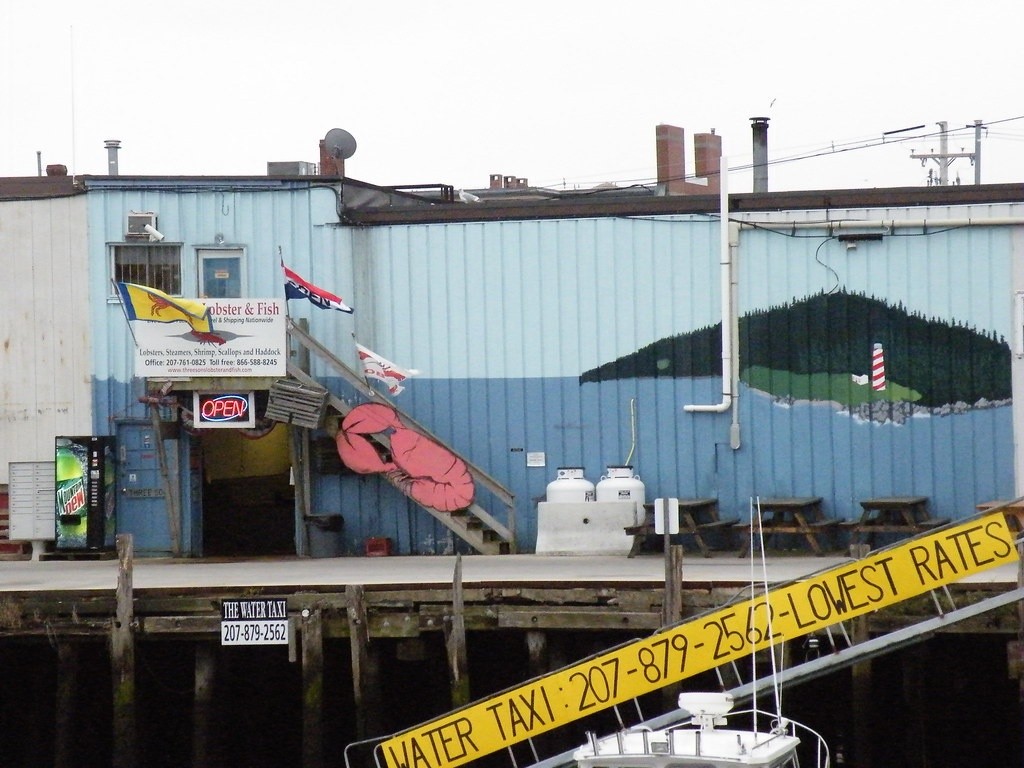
[324,128,356,161]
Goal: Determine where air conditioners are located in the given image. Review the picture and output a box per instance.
[126,209,158,237]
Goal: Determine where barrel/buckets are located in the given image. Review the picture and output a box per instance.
[595,466,645,528]
[546,466,595,503]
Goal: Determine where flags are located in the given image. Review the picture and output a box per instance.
[354,343,421,398]
[284,267,355,319]
[117,280,213,333]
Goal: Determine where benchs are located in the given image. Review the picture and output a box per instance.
[921,515,951,529]
[624,519,655,536]
[808,514,844,529]
[838,513,877,530]
[697,516,739,530]
[734,512,772,529]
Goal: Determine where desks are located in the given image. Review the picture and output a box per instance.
[845,495,933,557]
[975,498,1024,534]
[736,495,831,559]
[627,498,729,560]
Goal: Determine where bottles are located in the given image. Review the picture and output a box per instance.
[56,447,87,534]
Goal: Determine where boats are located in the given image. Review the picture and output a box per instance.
[503,491,834,768]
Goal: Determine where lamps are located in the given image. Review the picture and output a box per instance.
[141,223,165,243]
